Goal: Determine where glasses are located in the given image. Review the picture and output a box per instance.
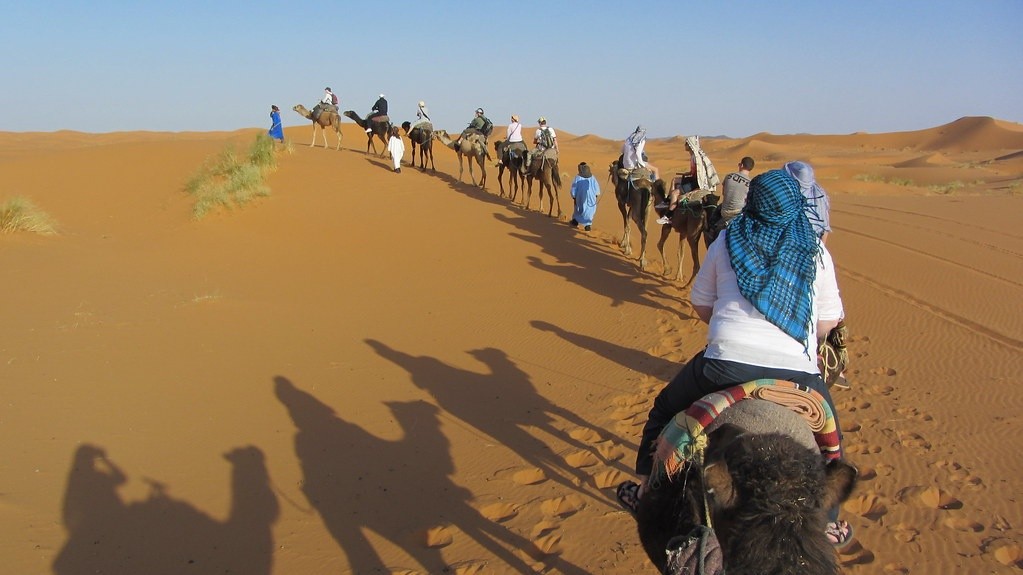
[739,163,741,166]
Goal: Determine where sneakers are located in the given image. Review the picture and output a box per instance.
[655,200,670,208]
[656,215,672,225]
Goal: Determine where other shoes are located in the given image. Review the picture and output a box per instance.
[585,225,591,231]
[523,170,531,176]
[833,375,849,389]
[570,221,579,229]
[495,161,503,167]
[365,128,372,133]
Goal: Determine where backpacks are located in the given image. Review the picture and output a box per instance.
[539,127,554,149]
[479,116,493,135]
[330,93,338,104]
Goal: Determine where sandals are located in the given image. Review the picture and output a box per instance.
[824,519,853,547]
[616,480,645,522]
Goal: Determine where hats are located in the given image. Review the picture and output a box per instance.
[325,87,331,92]
[475,108,484,114]
[379,94,384,97]
[512,115,518,122]
[418,101,424,106]
[537,117,546,122]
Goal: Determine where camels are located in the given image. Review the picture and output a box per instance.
[515,146,562,219]
[343,110,392,159]
[493,140,527,205]
[432,126,492,190]
[292,102,343,151]
[700,194,726,249]
[631,396,825,575]
[699,422,859,575]
[650,179,724,291]
[610,154,655,272]
[401,121,436,171]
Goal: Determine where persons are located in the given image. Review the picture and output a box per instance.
[782,160,833,246]
[267,105,284,143]
[621,126,668,198]
[495,115,527,167]
[569,162,600,231]
[403,101,430,137]
[655,135,720,224]
[615,170,853,547]
[322,87,339,113]
[721,157,754,222]
[522,117,559,175]
[365,94,387,133]
[388,126,404,173]
[454,108,488,148]
